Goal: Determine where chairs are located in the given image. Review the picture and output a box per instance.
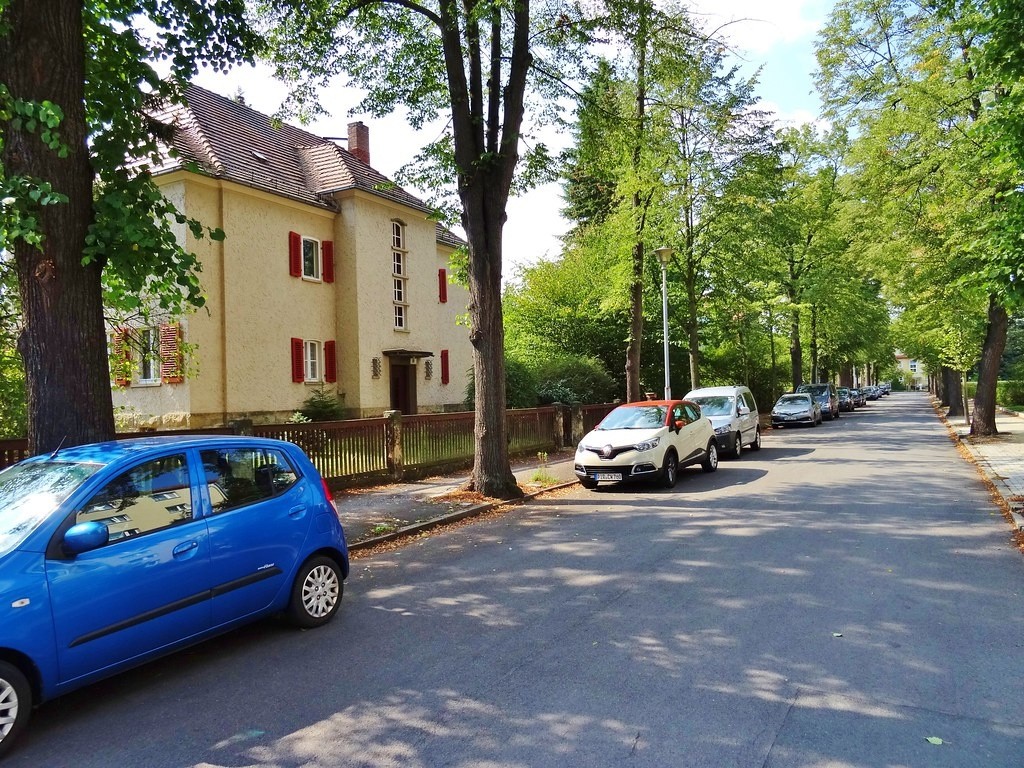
[255,463,286,498]
[151,473,184,521]
[106,473,140,499]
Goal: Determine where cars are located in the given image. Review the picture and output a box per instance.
[573,400,718,490]
[850,389,863,407]
[836,389,855,412]
[771,393,822,430]
[0,435,349,756]
[862,385,889,405]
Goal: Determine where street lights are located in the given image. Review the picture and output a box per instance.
[654,244,674,400]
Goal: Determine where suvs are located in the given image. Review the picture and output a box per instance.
[675,384,761,459]
[796,382,840,421]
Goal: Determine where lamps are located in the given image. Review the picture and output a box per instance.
[424,359,433,380]
[371,356,383,379]
